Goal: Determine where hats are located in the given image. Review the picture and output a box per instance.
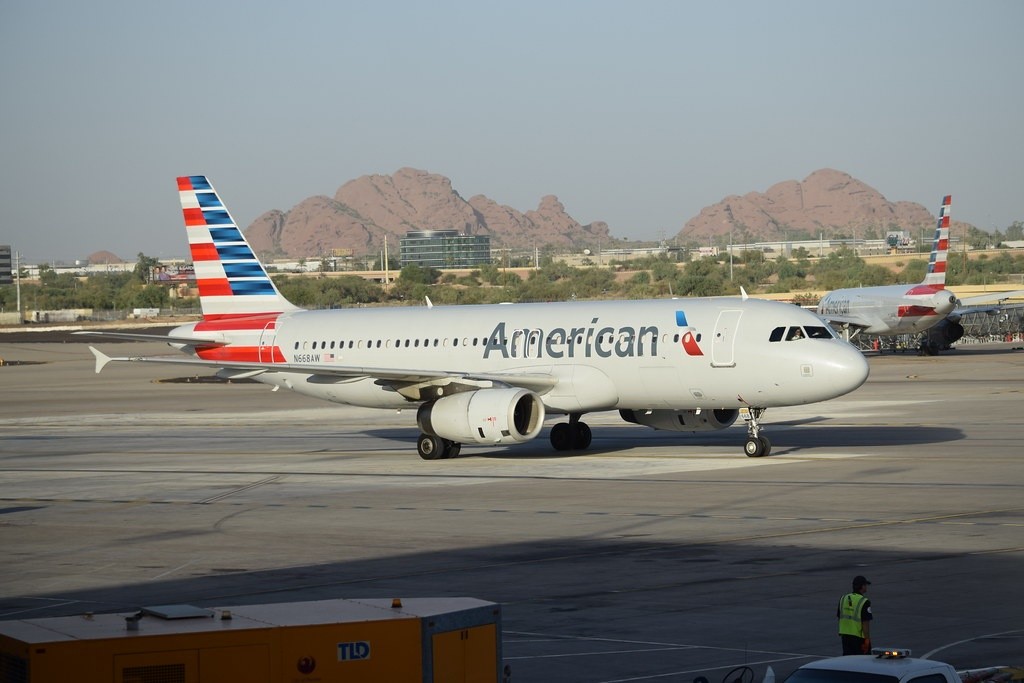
[852,576,871,585]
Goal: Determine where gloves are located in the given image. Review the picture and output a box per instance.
[862,639,871,654]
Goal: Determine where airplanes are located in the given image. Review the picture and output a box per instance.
[816,193,1024,351]
[67,173,870,460]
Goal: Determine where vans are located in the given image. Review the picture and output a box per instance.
[783,647,962,683]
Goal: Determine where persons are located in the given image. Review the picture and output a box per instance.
[838,575,873,657]
